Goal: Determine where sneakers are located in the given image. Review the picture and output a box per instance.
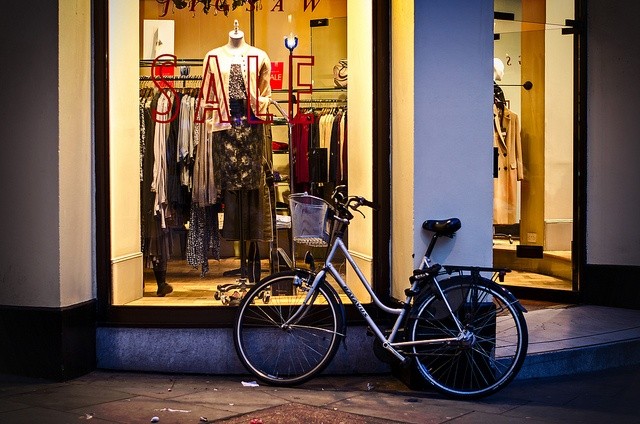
[157,282,173,297]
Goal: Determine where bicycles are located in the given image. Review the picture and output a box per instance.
[233,184,528,400]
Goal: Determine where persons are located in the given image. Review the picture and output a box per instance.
[494,57,506,107]
[201,30,273,192]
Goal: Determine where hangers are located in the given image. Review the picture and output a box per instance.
[141,74,203,102]
[494,87,518,121]
[295,98,347,118]
